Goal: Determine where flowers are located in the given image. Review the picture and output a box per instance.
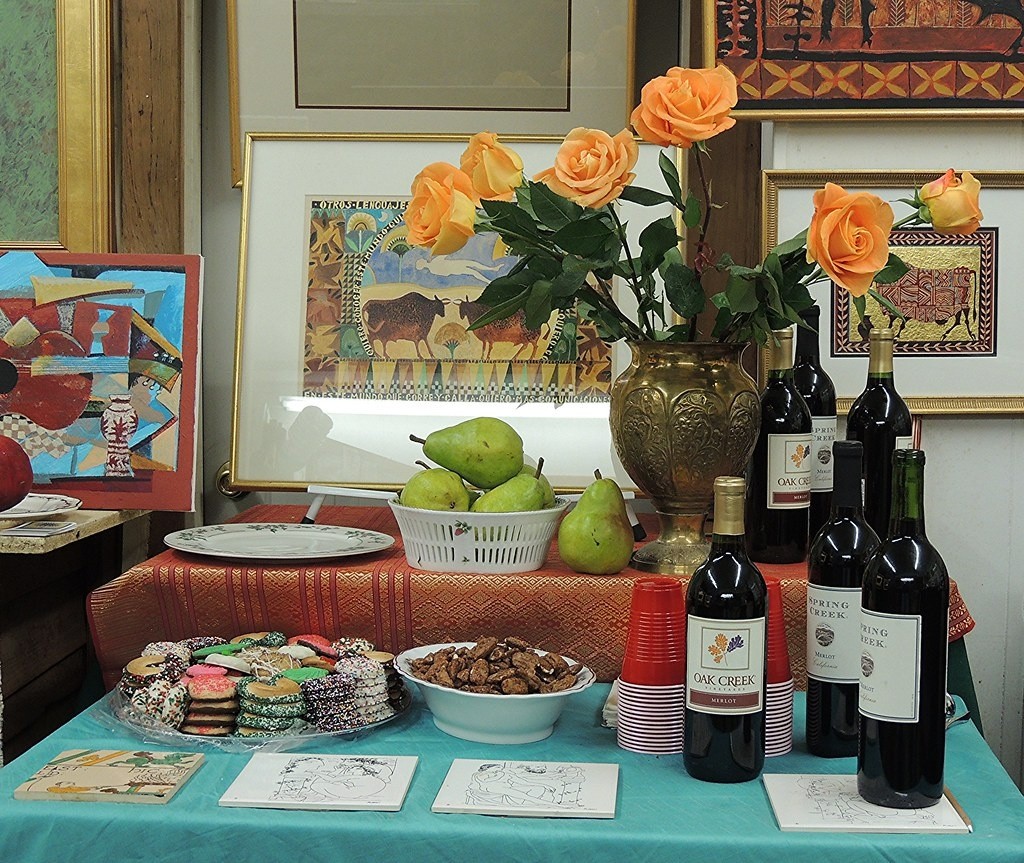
[403,66,982,341]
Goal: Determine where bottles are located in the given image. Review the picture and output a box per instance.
[805,440,880,761]
[857,447,950,810]
[792,304,836,536]
[845,328,914,541]
[744,325,811,565]
[683,476,765,784]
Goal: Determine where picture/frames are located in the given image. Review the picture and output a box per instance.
[228,131,691,498]
[0,0,113,258]
[758,171,1024,417]
[700,0,1024,121]
[228,0,635,187]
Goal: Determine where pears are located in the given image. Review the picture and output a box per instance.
[397,416,556,542]
[558,468,635,576]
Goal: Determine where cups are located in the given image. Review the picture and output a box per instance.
[762,576,795,757]
[615,576,685,755]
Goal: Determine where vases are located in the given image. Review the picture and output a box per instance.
[611,336,763,575]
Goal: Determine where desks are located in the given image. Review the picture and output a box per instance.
[87,500,983,733]
[0,681,1024,863]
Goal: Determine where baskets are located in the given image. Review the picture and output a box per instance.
[388,498,571,574]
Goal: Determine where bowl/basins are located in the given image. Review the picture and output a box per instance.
[392,642,596,745]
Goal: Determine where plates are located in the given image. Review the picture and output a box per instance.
[162,522,396,562]
[0,493,84,519]
[108,678,412,745]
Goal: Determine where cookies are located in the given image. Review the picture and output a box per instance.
[409,636,582,695]
[119,629,404,739]
